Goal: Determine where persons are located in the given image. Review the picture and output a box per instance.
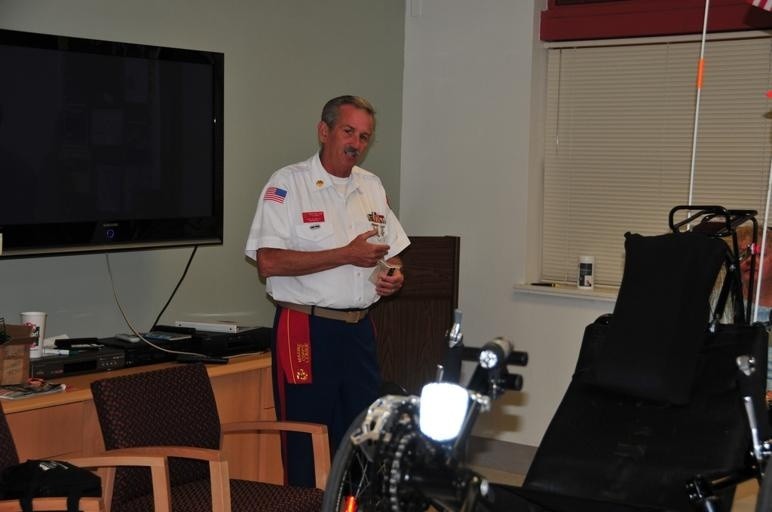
[244,95,411,489]
[728,226,772,405]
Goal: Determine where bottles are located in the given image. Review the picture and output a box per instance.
[577,254,596,291]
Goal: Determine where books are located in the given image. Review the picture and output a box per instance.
[145,331,193,341]
[0,382,66,400]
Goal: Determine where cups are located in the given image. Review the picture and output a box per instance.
[19,311,47,360]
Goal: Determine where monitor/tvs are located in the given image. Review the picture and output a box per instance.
[0,29,224,260]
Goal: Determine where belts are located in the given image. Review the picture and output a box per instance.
[277,299,369,323]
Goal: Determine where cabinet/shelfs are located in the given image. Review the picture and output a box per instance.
[367,235,459,396]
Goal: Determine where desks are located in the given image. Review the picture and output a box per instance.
[0,351,283,488]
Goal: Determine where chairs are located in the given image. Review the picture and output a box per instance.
[90,363,331,511]
[0,401,172,512]
